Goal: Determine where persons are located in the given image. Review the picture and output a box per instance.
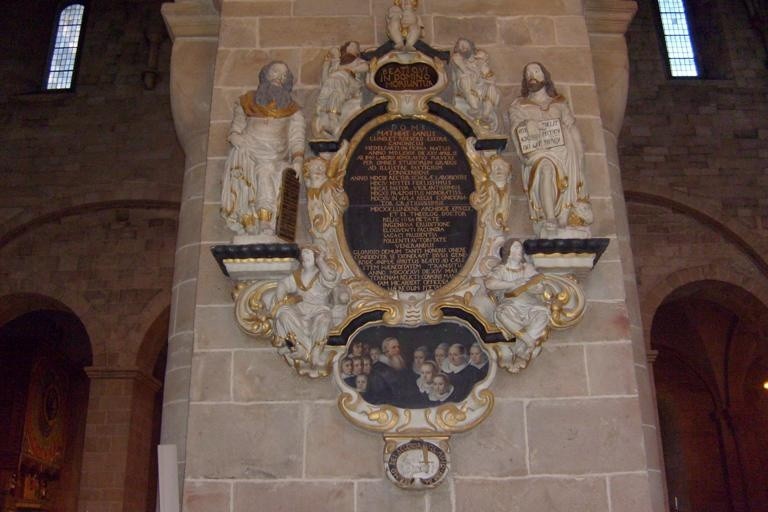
[219,0,591,430]
